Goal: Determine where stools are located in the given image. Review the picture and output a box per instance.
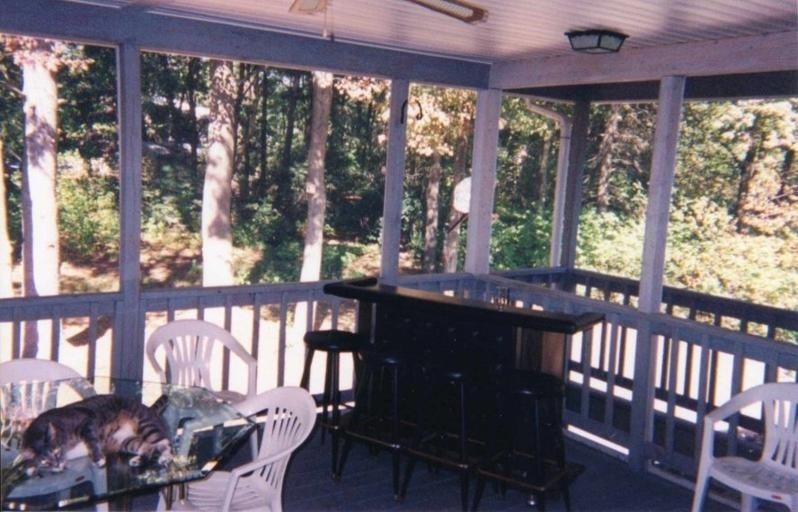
[470,371,571,512]
[301,330,379,481]
[335,339,434,500]
[399,353,491,511]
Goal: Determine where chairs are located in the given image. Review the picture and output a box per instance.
[147,321,258,463]
[0,359,109,511]
[156,385,318,512]
[691,382,798,512]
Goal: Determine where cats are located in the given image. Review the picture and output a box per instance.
[13,394,172,477]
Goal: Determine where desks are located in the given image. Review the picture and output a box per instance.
[323,277,606,473]
[0,376,259,510]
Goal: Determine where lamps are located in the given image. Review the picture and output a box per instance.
[567,28,629,55]
